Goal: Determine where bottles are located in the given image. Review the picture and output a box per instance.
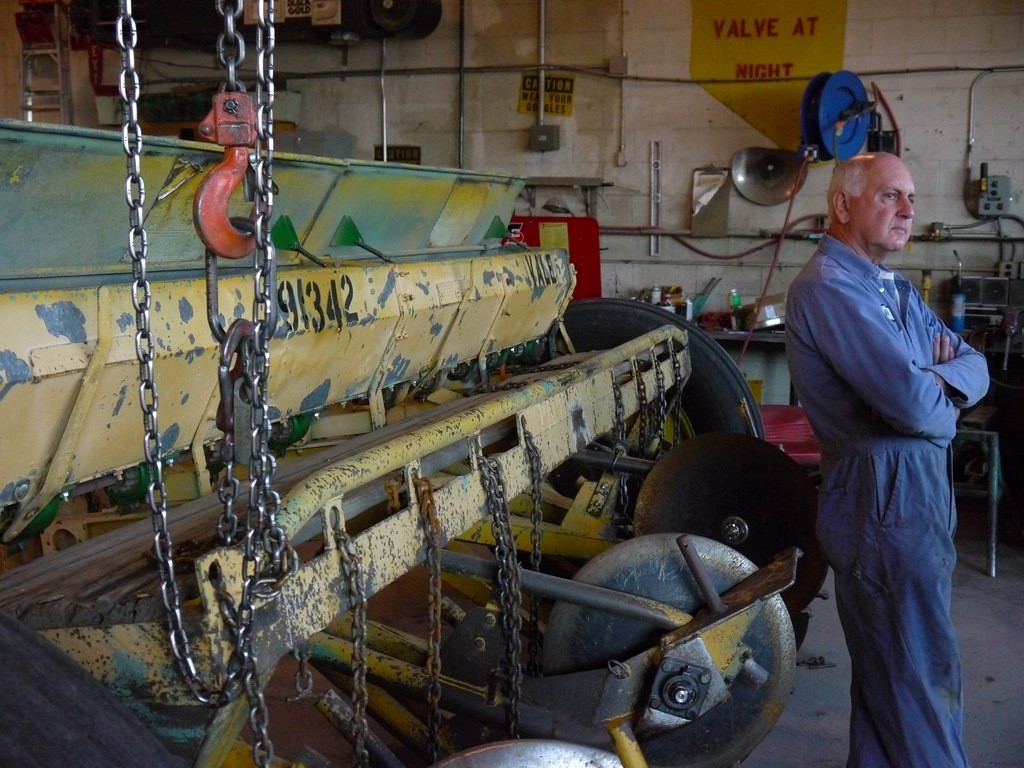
[730,288,744,330]
[651,283,661,304]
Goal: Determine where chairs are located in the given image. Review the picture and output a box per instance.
[761,405,820,467]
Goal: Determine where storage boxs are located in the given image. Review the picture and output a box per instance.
[278,131,355,158]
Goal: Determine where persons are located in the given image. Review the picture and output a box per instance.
[783,152,990,768]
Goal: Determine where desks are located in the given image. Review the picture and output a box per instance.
[704,329,796,405]
